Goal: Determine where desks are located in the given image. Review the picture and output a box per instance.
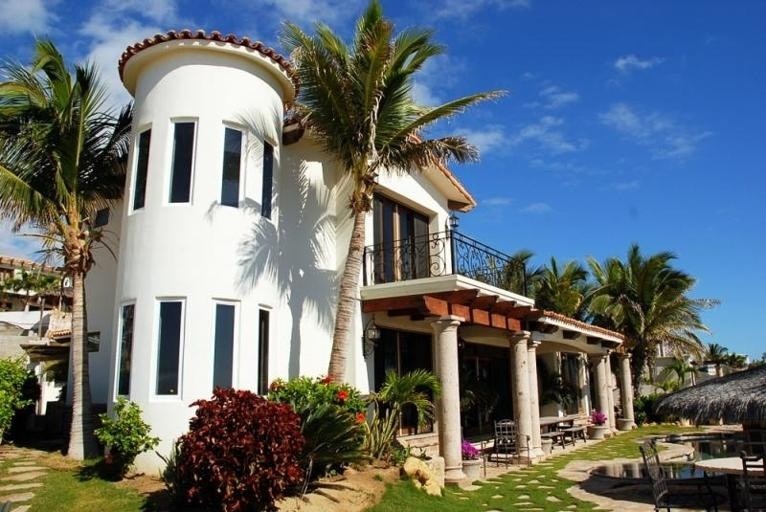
[497,414,581,447]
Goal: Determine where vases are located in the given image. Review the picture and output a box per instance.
[461,457,484,483]
[588,424,608,440]
[617,418,634,431]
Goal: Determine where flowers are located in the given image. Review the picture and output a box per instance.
[461,439,482,459]
[591,411,608,426]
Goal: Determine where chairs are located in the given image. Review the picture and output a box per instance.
[493,418,530,470]
[638,437,766,512]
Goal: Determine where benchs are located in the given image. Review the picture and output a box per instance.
[540,426,589,453]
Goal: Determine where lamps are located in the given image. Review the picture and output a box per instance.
[445,208,460,240]
[363,316,379,357]
